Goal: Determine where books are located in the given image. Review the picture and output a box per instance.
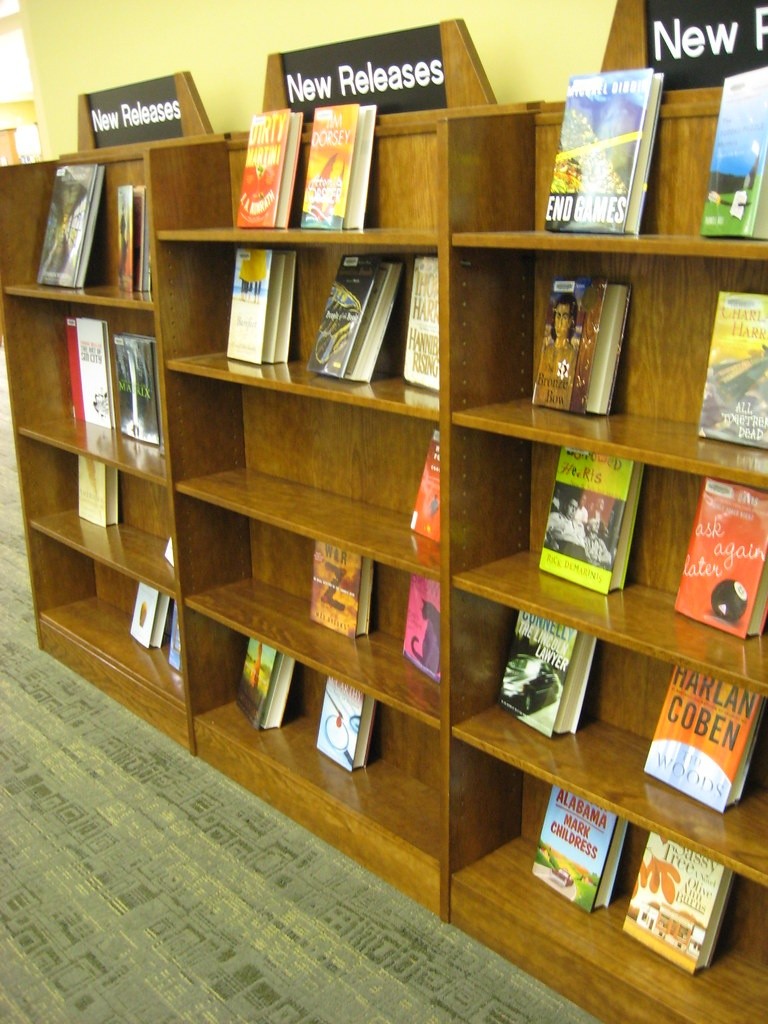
[236,637,295,731]
[701,68,767,240]
[403,575,441,682]
[237,104,378,231]
[317,676,376,773]
[532,785,630,913]
[675,478,768,639]
[539,446,644,595]
[698,292,768,449]
[498,610,597,738]
[532,279,631,416]
[623,831,733,974]
[311,541,374,640]
[36,163,182,672]
[227,248,295,365]
[307,253,406,383]
[411,429,440,544]
[544,68,663,235]
[644,665,765,812]
[404,257,440,393]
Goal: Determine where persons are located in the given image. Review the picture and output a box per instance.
[547,490,612,566]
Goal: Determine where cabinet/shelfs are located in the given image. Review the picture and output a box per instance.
[0,85,767,1024]
